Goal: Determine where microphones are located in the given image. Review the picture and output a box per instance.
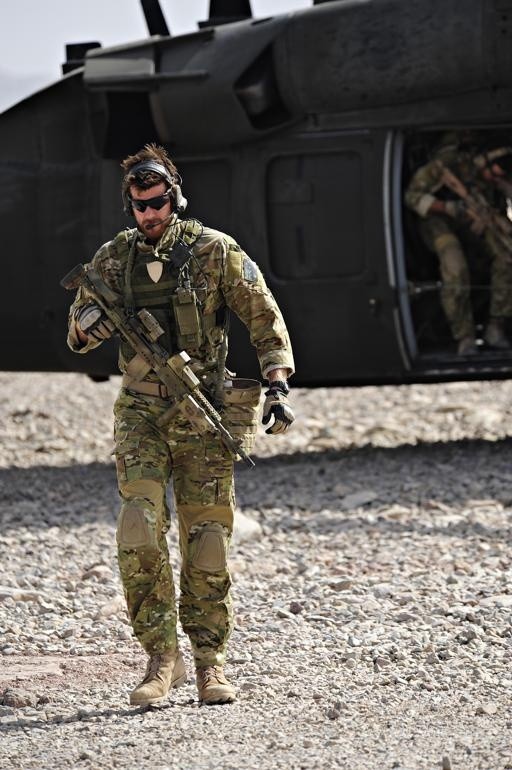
[144,211,175,230]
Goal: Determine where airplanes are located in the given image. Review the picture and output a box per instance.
[0,1,511,387]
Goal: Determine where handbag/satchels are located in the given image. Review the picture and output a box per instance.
[218,377,262,463]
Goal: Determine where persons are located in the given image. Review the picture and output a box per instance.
[400,134,511,359]
[63,141,298,707]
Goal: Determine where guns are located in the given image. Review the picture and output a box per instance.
[60,264,257,469]
[441,170,512,251]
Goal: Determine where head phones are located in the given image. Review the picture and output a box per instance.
[121,161,189,218]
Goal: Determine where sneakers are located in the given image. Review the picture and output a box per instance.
[483,318,510,350]
[196,664,236,705]
[456,336,481,359]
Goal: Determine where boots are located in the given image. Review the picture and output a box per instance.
[130,649,187,706]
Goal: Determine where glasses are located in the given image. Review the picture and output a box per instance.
[128,191,170,214]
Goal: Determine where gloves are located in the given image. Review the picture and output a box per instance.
[73,303,117,343]
[261,381,296,436]
